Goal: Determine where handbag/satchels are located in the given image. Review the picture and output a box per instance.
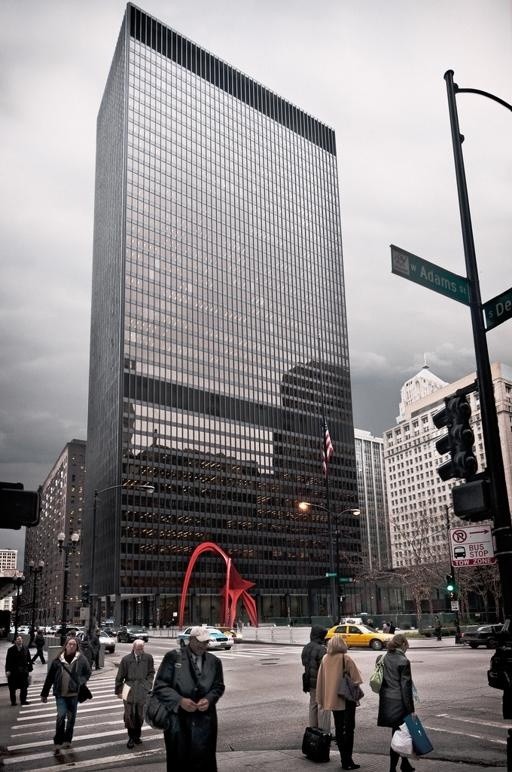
[390,712,435,761]
[142,691,183,734]
[77,684,93,704]
[368,652,386,694]
[302,671,311,693]
[336,670,365,708]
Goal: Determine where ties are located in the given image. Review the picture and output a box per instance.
[136,655,141,663]
[193,656,201,675]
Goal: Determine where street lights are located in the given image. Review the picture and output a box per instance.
[12,570,27,642]
[88,482,155,653]
[58,532,80,649]
[298,499,361,618]
[28,559,42,644]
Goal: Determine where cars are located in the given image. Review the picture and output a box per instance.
[323,617,397,651]
[118,625,149,643]
[485,649,511,691]
[177,624,233,651]
[10,619,118,654]
[459,623,504,648]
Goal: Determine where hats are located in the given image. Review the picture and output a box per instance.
[188,625,215,644]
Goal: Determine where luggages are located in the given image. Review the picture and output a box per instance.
[301,707,333,765]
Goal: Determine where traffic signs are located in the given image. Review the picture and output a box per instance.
[390,245,469,307]
[483,289,512,332]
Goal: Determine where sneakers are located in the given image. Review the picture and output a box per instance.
[53,740,72,757]
[95,666,103,670]
[400,760,416,772]
[10,699,31,707]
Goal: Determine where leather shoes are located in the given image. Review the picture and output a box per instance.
[341,761,362,769]
[127,736,144,749]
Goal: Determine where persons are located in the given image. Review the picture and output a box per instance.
[302,624,331,734]
[31,630,47,665]
[40,637,91,756]
[376,634,418,772]
[365,617,396,635]
[114,638,156,749]
[316,634,365,770]
[80,636,96,668]
[4,636,34,705]
[433,613,442,641]
[90,630,102,670]
[152,626,225,772]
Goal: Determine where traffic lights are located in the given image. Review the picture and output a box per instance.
[1,485,39,530]
[446,575,454,593]
[431,405,456,484]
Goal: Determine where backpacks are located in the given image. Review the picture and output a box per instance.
[486,645,512,691]
